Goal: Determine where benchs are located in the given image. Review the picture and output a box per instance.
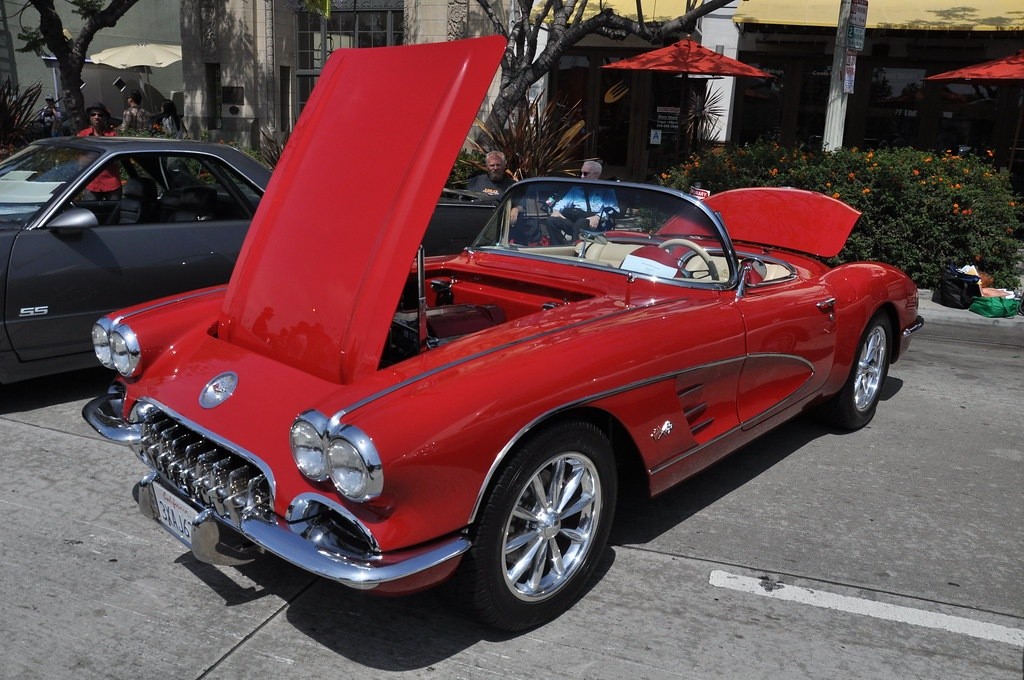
[159,188,261,220]
[575,240,790,283]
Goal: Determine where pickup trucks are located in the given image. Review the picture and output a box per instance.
[1,137,501,389]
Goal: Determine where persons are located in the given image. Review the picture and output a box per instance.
[547,161,620,247]
[120,89,151,132]
[39,94,62,125]
[77,102,138,201]
[466,150,527,245]
[161,99,189,140]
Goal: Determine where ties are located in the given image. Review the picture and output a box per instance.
[583,189,592,212]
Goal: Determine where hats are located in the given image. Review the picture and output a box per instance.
[86,102,111,119]
[45,94,53,101]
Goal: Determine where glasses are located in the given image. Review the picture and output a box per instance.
[489,164,502,167]
[89,112,101,117]
[127,97,131,99]
[580,171,592,177]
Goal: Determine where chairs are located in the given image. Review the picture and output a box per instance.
[537,177,620,238]
[163,186,217,223]
[104,176,158,226]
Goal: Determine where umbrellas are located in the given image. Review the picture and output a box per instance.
[91,43,182,88]
[923,50,1023,176]
[602,33,776,162]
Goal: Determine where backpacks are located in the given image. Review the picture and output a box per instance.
[931,265,982,310]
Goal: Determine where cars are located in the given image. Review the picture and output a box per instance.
[80,35,928,638]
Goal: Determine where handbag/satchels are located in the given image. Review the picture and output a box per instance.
[970,296,1022,319]
